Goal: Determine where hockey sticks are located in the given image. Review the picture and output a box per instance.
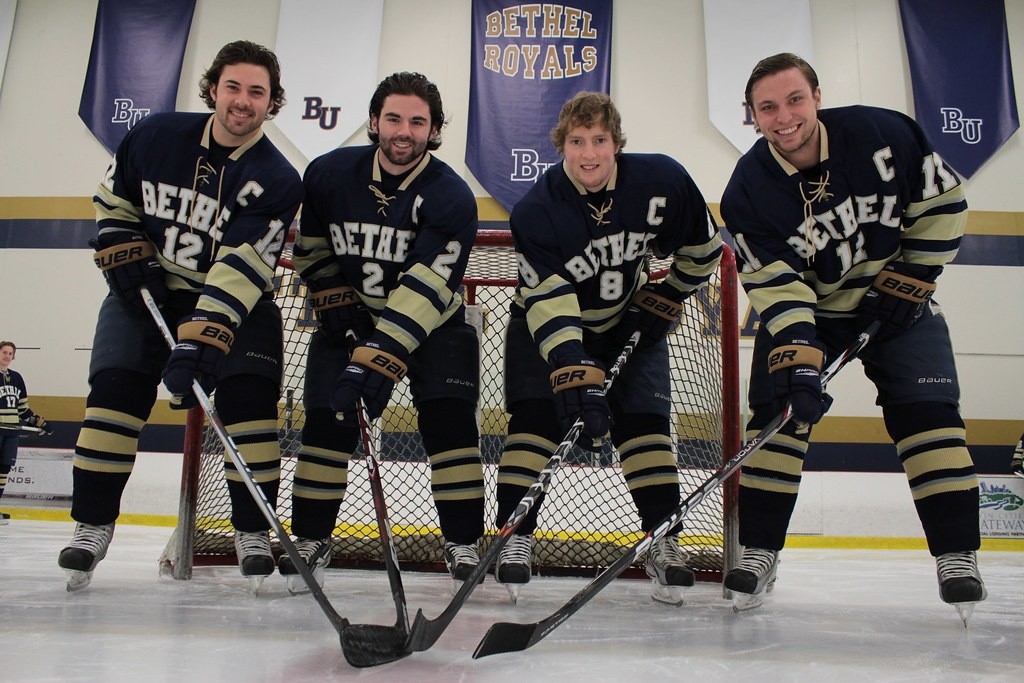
[470,319,884,659]
[404,331,640,653]
[87,235,349,634]
[339,329,414,669]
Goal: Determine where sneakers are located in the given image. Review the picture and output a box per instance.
[935,550,988,628]
[494,535,532,605]
[724,547,781,613]
[278,535,331,596]
[443,542,486,598]
[234,529,275,596]
[646,533,696,607]
[57,522,116,592]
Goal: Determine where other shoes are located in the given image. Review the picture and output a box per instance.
[0,513,10,525]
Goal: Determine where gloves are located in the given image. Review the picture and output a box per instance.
[308,279,376,344]
[863,260,944,342]
[767,334,834,424]
[623,283,683,348]
[548,355,609,453]
[1011,458,1024,475]
[87,231,167,312]
[34,415,56,433]
[161,310,240,410]
[330,337,410,428]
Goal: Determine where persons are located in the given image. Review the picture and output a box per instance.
[278,70,486,597]
[57,40,303,591]
[495,91,724,606]
[719,52,988,628]
[0,340,55,525]
